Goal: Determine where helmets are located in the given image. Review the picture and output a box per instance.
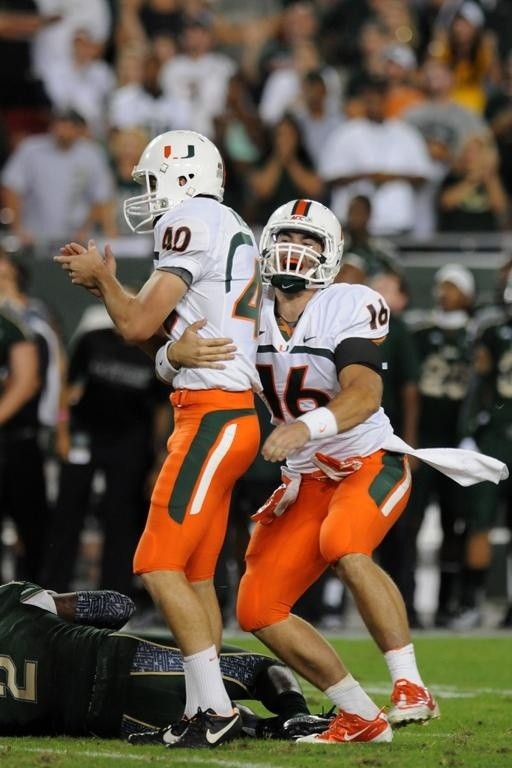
[122,130,225,235]
[258,197,345,292]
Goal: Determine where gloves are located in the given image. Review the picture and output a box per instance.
[249,465,302,525]
[311,451,364,481]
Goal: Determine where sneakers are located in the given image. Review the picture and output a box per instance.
[295,709,394,745]
[382,678,441,725]
[128,701,245,747]
[242,715,281,738]
[278,705,337,740]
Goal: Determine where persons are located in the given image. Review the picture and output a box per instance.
[0,129,509,750]
[0,1,512,636]
